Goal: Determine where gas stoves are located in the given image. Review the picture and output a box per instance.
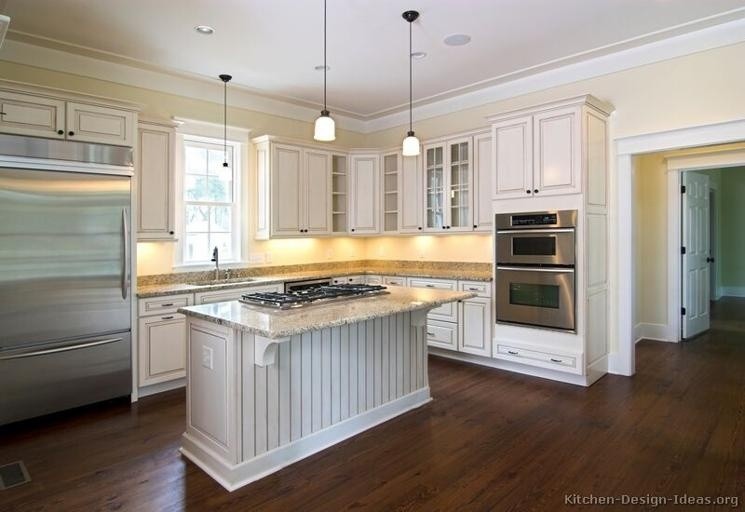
[238,282,387,310]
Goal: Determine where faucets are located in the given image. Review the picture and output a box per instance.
[209,245,219,281]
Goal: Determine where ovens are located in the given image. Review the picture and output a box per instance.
[494,209,579,333]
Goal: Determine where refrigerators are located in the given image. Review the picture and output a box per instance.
[1,131,136,425]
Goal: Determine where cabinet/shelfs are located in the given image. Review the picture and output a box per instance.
[0,80,138,145]
[194,283,285,303]
[333,275,494,362]
[494,322,588,386]
[179,308,433,494]
[138,293,194,398]
[134,119,179,241]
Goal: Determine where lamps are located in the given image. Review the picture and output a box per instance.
[401,10,421,157]
[219,74,233,177]
[313,0,336,142]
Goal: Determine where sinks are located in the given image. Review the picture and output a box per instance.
[185,278,255,287]
